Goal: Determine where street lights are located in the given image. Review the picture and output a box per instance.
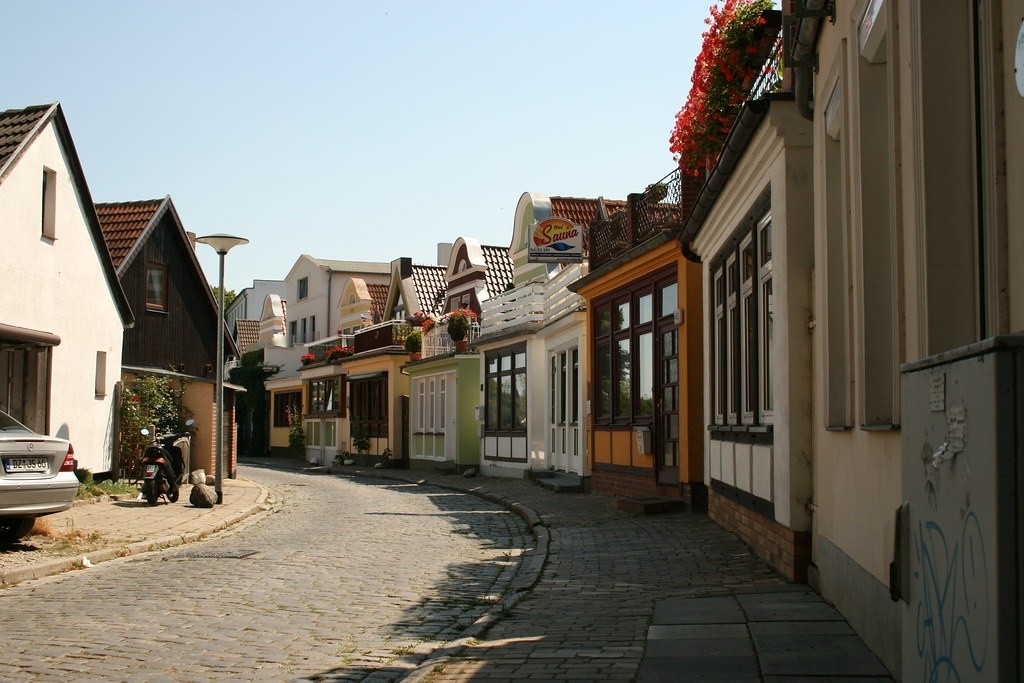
[193,233,251,504]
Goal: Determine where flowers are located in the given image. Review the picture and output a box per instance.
[668,0,779,179]
[285,401,306,448]
[434,307,477,327]
[422,320,436,335]
[404,312,432,324]
[324,345,355,362]
[300,353,316,364]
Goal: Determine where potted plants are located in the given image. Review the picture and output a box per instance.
[446,315,471,352]
[405,330,422,361]
[646,182,667,203]
[609,207,627,223]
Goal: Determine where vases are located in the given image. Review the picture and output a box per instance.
[333,354,349,360]
[410,321,423,326]
[706,10,782,159]
[303,361,314,367]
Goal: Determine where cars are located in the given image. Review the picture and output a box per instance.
[0,407,80,544]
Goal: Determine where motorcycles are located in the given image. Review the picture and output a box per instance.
[137,419,195,507]
